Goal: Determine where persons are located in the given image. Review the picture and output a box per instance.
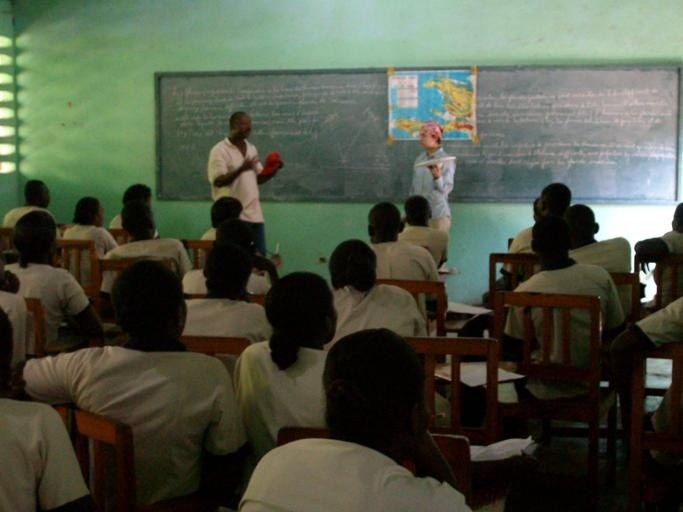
[238,327,469,508]
[207,111,277,264]
[233,272,338,451]
[1,311,95,508]
[3,180,277,356]
[367,201,447,312]
[323,239,426,347]
[16,259,244,507]
[401,196,448,268]
[501,183,683,433]
[408,122,455,240]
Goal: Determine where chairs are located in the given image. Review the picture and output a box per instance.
[0,222,682,512]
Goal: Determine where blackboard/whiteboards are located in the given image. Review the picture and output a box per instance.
[155,66,682,205]
